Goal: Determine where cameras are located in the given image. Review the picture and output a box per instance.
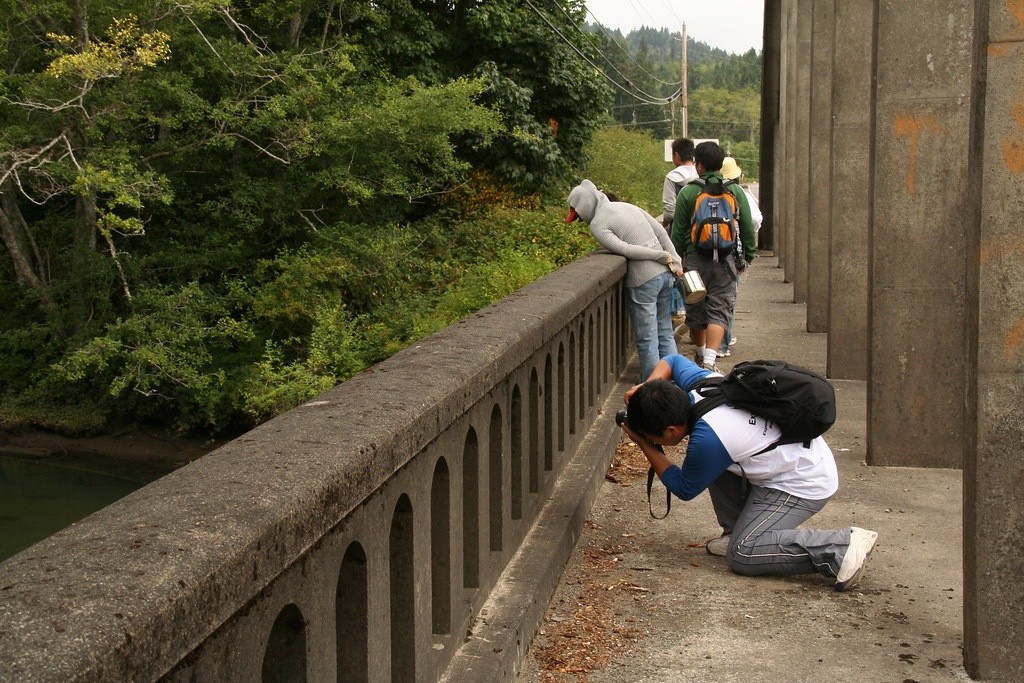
[616,407,631,429]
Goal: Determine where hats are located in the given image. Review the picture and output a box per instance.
[567,207,578,222]
[719,157,741,180]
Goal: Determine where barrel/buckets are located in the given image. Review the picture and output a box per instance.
[675,270,707,304]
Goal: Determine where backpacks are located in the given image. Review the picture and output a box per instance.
[687,360,836,458]
[688,179,740,263]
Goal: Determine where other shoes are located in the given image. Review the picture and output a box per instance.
[694,353,704,367]
[730,336,738,346]
[716,349,730,357]
[699,361,726,376]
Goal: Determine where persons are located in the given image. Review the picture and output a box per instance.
[671,142,756,372]
[565,179,683,385]
[621,354,880,589]
[661,138,702,336]
[720,157,762,346]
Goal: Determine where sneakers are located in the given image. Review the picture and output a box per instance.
[833,526,879,592]
[705,534,730,557]
